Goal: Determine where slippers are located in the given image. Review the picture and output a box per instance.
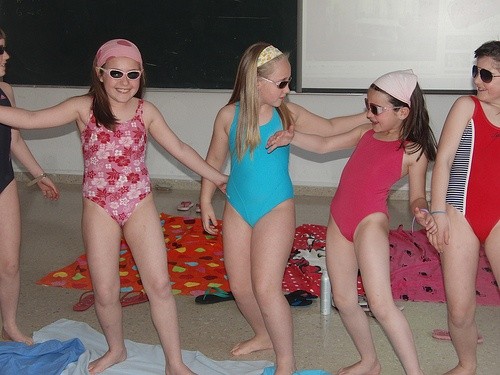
[195,286,234,304]
[120,290,149,307]
[431,327,484,343]
[284,295,312,306]
[289,290,318,300]
[73,291,94,311]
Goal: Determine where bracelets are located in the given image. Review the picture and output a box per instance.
[412,209,429,235]
[27,173,46,188]
[431,211,447,216]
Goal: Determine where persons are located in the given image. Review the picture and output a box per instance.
[265,68,437,375]
[200,43,373,375]
[0,30,59,345]
[1,39,230,374]
[431,42,500,375]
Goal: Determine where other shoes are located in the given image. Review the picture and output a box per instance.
[369,304,404,316]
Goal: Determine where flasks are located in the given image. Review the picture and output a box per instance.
[320,271,332,315]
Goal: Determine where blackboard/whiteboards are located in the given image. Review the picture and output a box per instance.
[0,0,302,94]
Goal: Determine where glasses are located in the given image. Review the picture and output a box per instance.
[365,98,410,116]
[0,45,8,55]
[257,76,293,89]
[99,68,144,80]
[472,65,500,83]
